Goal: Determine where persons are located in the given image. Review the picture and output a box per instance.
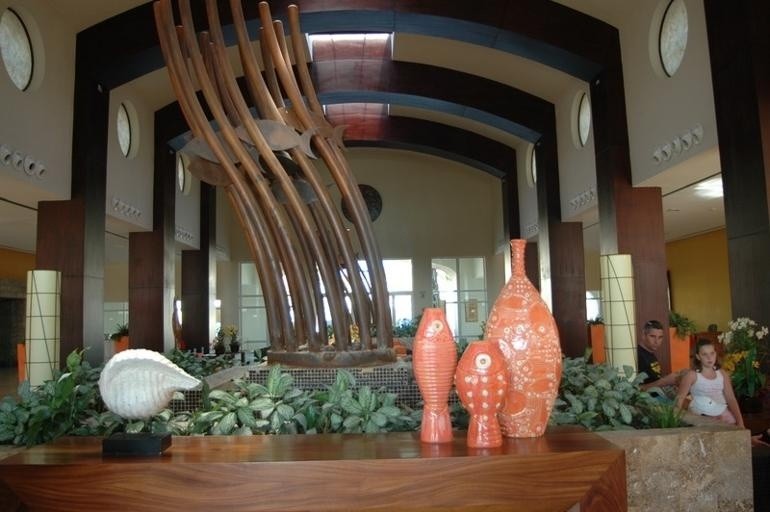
[674,338,745,428]
[638,321,692,410]
[688,396,770,448]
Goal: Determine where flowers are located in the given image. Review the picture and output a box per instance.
[719,317,769,397]
[212,323,241,342]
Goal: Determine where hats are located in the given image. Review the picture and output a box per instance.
[688,396,728,416]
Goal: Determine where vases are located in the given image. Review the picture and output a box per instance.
[215,341,239,353]
[740,398,765,414]
[414,235,565,449]
